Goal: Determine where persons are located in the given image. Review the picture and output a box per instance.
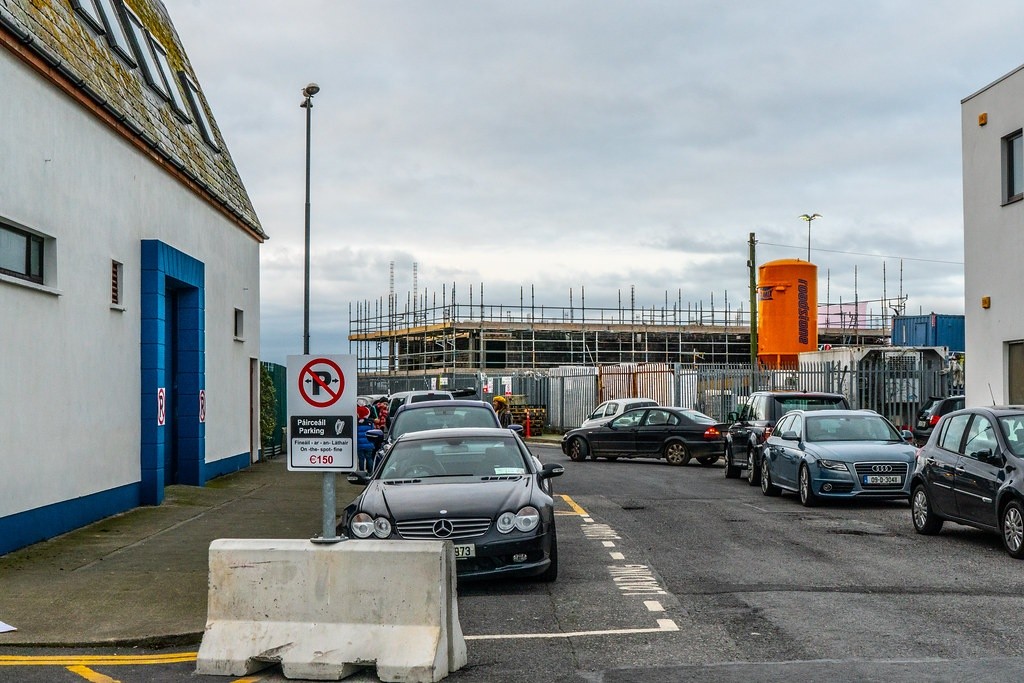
[357,397,393,476]
[492,395,514,428]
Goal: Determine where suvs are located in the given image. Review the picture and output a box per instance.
[723,390,853,486]
[913,393,965,445]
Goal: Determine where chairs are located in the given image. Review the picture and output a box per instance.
[461,411,488,426]
[401,449,447,477]
[986,423,1011,438]
[477,446,517,474]
[399,410,428,431]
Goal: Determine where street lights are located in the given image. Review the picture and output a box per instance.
[799,213,822,263]
[302,81,322,354]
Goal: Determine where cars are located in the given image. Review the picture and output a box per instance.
[581,397,661,429]
[909,404,1024,560]
[561,405,733,466]
[338,423,566,584]
[355,390,525,462]
[759,409,920,508]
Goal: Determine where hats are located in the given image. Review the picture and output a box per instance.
[357,406,370,419]
[379,396,389,403]
[492,396,506,403]
